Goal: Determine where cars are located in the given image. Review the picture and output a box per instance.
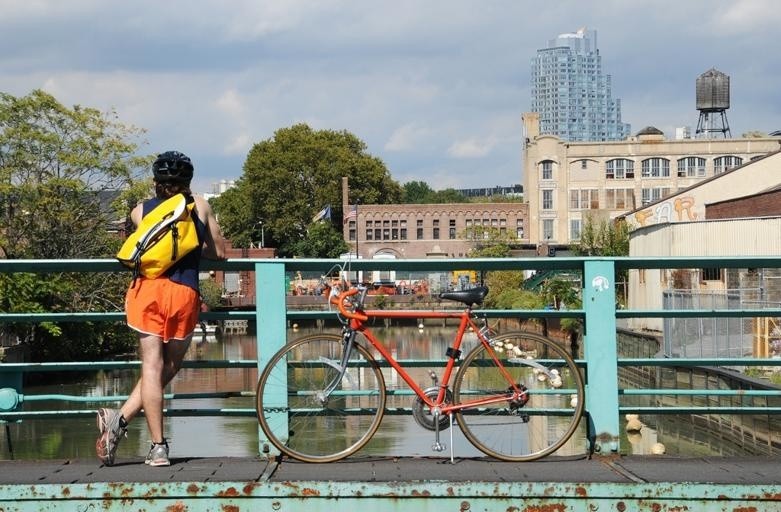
[351,277,414,296]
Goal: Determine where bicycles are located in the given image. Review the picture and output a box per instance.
[256,264,584,463]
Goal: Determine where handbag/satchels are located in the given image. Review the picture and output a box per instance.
[116,192,201,278]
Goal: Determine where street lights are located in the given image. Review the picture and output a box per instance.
[251,219,264,247]
[354,195,361,284]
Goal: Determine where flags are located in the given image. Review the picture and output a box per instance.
[312,203,331,225]
[343,203,357,225]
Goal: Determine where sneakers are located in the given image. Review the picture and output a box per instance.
[96,408,129,466]
[145,442,171,467]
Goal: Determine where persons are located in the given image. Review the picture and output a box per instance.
[291,272,474,296]
[97,150,226,468]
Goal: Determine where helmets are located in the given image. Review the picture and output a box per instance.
[152,151,194,184]
